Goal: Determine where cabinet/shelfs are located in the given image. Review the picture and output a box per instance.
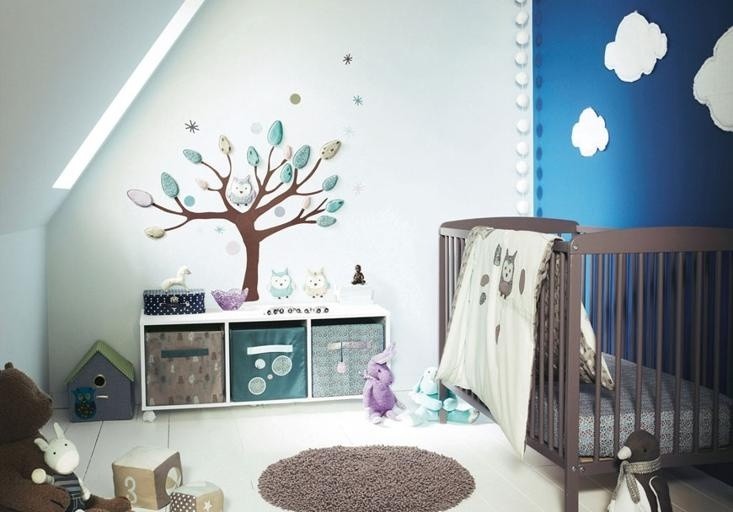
[140,303,392,422]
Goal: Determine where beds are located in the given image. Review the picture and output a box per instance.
[439,217,733,512]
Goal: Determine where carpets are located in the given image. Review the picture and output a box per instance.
[257,445,476,512]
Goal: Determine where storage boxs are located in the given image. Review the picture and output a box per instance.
[144,331,224,405]
[229,326,308,401]
[311,324,385,396]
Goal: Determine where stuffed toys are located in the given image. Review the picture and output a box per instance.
[362,341,407,425]
[0,362,131,512]
[31,423,91,512]
[604,430,672,512]
[406,368,479,428]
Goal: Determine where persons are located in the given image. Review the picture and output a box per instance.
[352,264,367,286]
